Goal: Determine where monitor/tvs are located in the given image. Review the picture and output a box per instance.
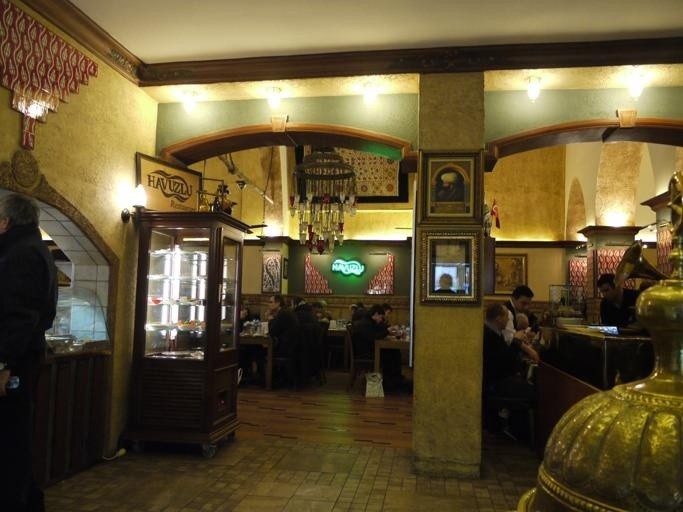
[589,325,620,337]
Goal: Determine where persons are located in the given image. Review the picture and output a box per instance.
[1,192,60,512]
[481,286,544,447]
[436,179,461,202]
[433,274,458,294]
[597,274,639,330]
[241,293,409,392]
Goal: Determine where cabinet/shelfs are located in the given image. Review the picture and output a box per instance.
[120,213,242,457]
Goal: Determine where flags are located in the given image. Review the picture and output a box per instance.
[490,200,500,228]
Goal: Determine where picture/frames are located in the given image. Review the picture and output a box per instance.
[420,229,485,305]
[492,253,528,296]
[416,147,485,226]
[293,144,409,204]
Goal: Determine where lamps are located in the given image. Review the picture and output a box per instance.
[290,145,359,256]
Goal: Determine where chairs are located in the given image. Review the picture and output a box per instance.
[341,325,373,391]
[287,319,349,391]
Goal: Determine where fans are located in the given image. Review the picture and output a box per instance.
[235,179,267,234]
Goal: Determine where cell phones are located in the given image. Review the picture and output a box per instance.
[524,327,532,334]
[5,376,19,388]
